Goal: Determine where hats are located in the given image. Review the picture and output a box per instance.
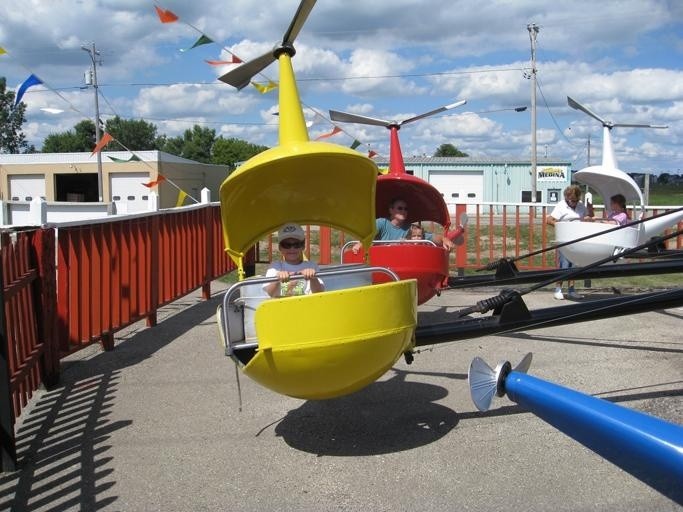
[279,223,306,242]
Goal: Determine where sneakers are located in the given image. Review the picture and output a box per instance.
[567,293,584,300]
[554,292,564,300]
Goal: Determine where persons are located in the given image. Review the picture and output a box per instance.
[351,198,455,255]
[406,224,425,245]
[545,183,595,300]
[262,223,325,297]
[592,194,631,225]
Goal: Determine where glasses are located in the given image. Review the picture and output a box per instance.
[392,206,407,211]
[280,242,302,249]
